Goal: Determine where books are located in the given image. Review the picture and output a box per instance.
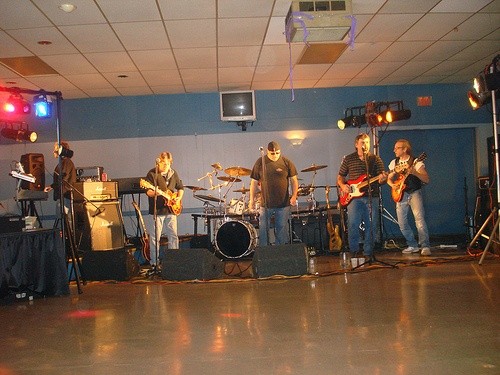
[24,217,37,229]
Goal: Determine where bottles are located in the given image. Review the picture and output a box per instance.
[308,246,318,275]
[102,170,108,182]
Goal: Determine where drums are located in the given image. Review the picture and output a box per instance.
[228,198,246,220]
[248,192,260,214]
[214,219,258,259]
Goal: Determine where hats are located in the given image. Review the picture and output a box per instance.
[268,141,280,151]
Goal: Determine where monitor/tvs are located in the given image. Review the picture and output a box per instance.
[219,90,256,122]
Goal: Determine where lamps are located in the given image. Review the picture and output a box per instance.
[1,87,53,143]
[467,71,500,110]
[337,101,411,130]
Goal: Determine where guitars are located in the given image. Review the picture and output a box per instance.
[340,163,410,207]
[324,185,342,252]
[131,202,160,261]
[138,178,183,216]
[392,152,427,203]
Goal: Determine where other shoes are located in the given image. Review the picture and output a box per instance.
[146,269,159,276]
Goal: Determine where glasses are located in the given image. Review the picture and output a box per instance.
[270,152,280,155]
[161,162,170,166]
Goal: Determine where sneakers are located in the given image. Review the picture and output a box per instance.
[403,246,419,253]
[421,247,431,255]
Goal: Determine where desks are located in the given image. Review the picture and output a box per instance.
[0,229,69,301]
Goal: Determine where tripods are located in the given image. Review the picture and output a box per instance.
[351,152,398,271]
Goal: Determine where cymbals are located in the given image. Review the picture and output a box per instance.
[196,194,224,202]
[300,165,328,172]
[234,189,250,192]
[183,185,208,191]
[225,167,252,176]
[217,176,242,182]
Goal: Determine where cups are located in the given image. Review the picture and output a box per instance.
[351,258,357,268]
[359,258,365,267]
[16,290,33,301]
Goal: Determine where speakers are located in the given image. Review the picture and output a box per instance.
[20,153,45,192]
[81,250,141,281]
[253,242,309,278]
[487,134,500,188]
[162,249,225,281]
[474,195,500,245]
[72,201,125,251]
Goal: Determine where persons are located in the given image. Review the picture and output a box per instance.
[336,134,387,262]
[249,141,298,246]
[387,139,431,255]
[44,142,75,230]
[145,152,183,274]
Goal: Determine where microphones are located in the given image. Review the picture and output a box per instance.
[362,146,367,152]
[92,209,105,217]
